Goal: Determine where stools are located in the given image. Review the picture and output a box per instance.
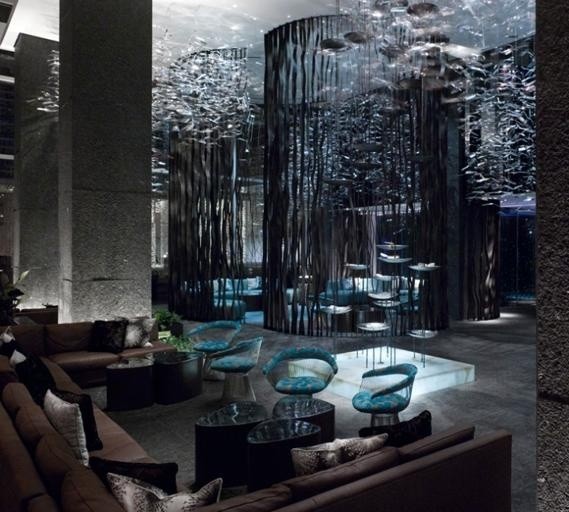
[356,322,393,338]
[311,305,352,336]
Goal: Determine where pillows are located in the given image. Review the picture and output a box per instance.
[358,409,434,442]
[43,388,90,468]
[123,317,154,349]
[105,474,222,512]
[89,319,124,352]
[291,433,390,477]
[55,385,104,454]
[90,456,178,497]
[11,358,60,408]
[248,278,259,290]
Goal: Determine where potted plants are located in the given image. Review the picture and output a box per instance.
[153,311,181,339]
[167,335,192,361]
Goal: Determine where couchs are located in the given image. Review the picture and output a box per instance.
[180,276,301,322]
[318,277,427,306]
[1,321,513,511]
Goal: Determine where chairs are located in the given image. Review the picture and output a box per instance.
[353,362,417,425]
[188,320,241,380]
[261,345,338,396]
[209,337,261,403]
